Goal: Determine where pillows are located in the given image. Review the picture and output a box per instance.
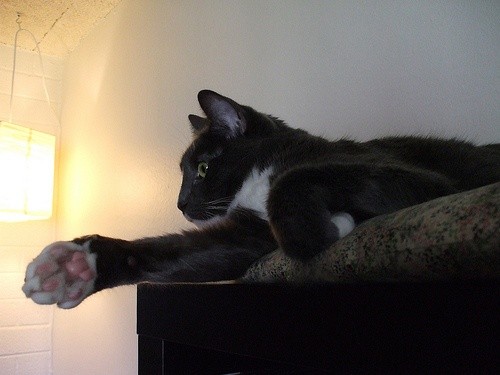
[236,183,500,288]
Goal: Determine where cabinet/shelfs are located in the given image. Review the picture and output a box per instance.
[136,275,500,375]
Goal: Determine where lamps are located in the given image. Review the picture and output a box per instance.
[0,29,56,224]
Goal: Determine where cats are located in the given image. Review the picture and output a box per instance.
[23,90,498,309]
